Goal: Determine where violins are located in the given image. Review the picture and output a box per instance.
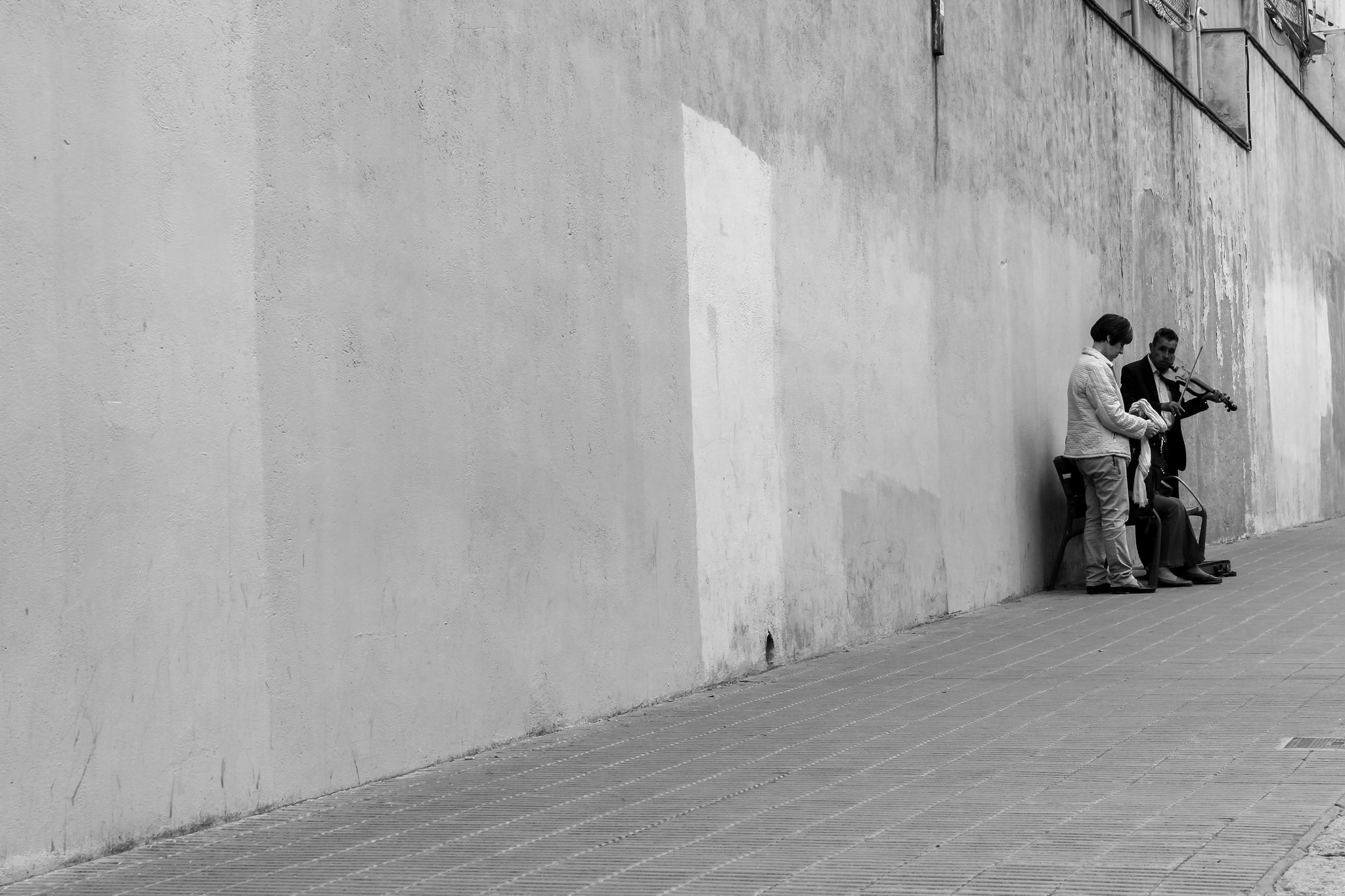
[1160,357,1237,413]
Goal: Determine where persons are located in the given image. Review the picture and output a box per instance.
[1125,449,1223,588]
[1064,313,1160,595]
[1119,327,1227,500]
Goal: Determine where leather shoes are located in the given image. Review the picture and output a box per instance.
[1153,574,1194,588]
[1086,582,1112,595]
[1181,569,1222,584]
[1112,580,1157,593]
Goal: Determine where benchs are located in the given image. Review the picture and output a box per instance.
[1052,455,1208,590]
